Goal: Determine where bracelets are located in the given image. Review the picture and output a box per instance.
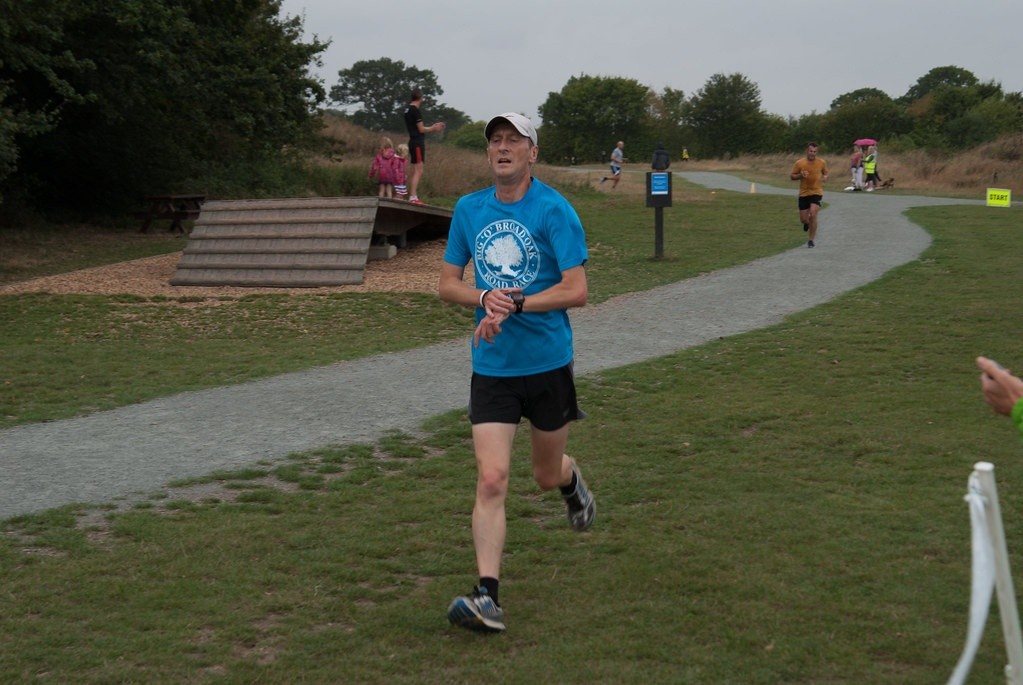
[479,290,491,307]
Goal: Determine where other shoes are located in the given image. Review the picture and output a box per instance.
[600,177,606,183]
[409,197,428,207]
[853,188,861,191]
[866,186,875,192]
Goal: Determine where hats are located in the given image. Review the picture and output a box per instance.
[484,112,537,146]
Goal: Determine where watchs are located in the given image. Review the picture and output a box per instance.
[510,293,525,313]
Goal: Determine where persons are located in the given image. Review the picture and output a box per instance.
[601,141,624,188]
[682,146,689,161]
[439,112,597,636]
[403,90,446,204]
[790,143,829,248]
[850,144,863,191]
[367,137,409,199]
[976,357,1023,433]
[861,146,881,188]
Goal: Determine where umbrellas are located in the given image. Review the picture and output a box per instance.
[853,139,877,145]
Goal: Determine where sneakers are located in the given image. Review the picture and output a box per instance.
[561,457,596,531]
[804,223,809,231]
[807,241,814,247]
[447,584,506,633]
